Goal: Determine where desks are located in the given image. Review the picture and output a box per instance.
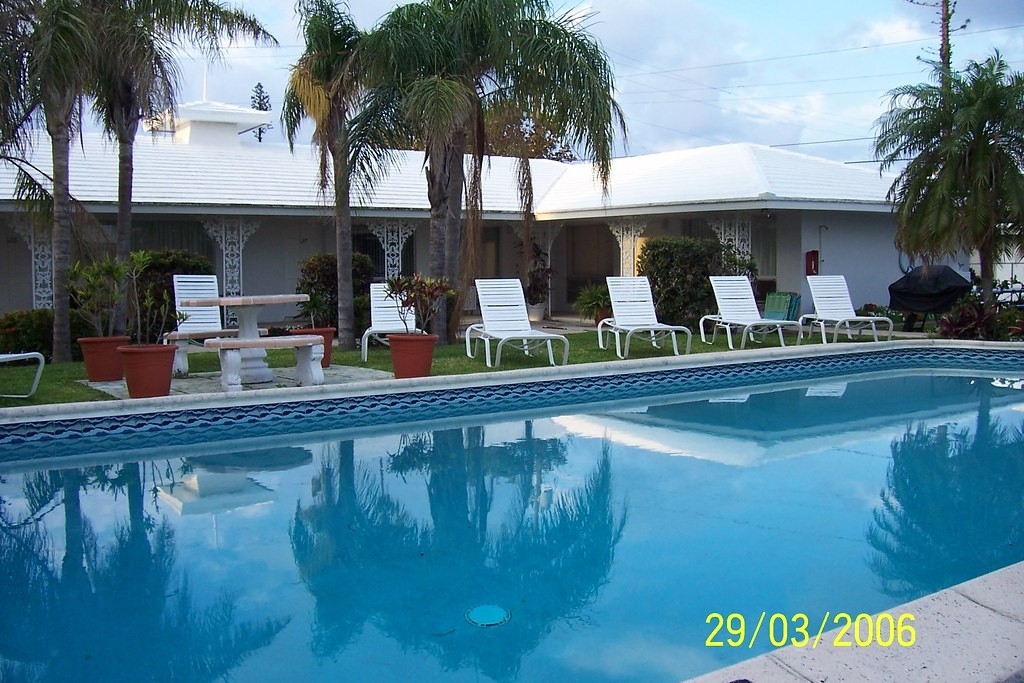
[180,294,310,384]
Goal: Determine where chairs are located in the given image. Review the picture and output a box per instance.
[466,279,569,369]
[173,275,222,361]
[361,283,429,362]
[598,277,692,360]
[798,275,893,343]
[699,276,802,351]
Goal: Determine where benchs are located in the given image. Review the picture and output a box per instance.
[163,329,268,379]
[204,335,324,392]
[0,352,45,398]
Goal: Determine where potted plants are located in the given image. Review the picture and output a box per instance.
[524,244,556,322]
[287,255,338,368]
[62,252,140,381]
[384,272,455,379]
[110,252,189,398]
[572,281,613,326]
